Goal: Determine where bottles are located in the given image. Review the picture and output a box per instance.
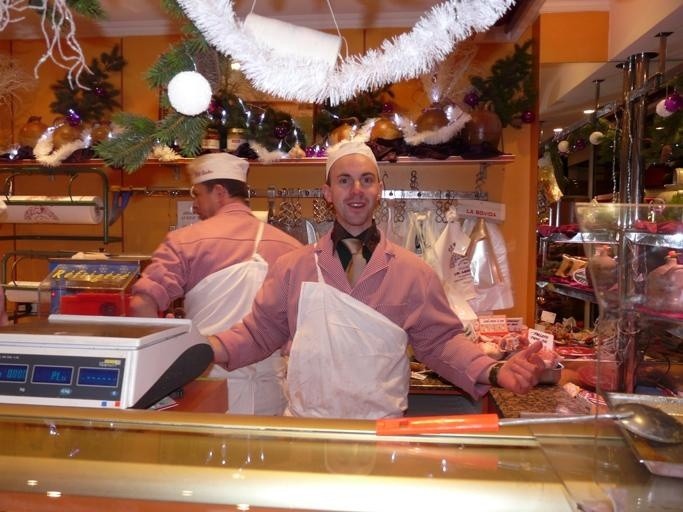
[328,97,503,152]
[585,248,617,288]
[645,257,682,312]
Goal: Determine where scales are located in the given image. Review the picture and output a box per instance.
[0,314,218,410]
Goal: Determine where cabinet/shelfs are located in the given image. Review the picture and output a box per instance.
[535,59,682,391]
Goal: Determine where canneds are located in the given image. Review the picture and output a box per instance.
[201,128,221,154]
[226,127,247,153]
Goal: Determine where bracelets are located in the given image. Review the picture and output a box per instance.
[489,363,504,387]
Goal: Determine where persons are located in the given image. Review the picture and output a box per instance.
[201,140,545,419]
[128,153,307,416]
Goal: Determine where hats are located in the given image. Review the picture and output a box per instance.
[186,151,250,185]
[325,140,381,178]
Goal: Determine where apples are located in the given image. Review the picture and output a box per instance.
[369,119,403,140]
[327,122,353,145]
[414,110,448,132]
[462,110,503,152]
